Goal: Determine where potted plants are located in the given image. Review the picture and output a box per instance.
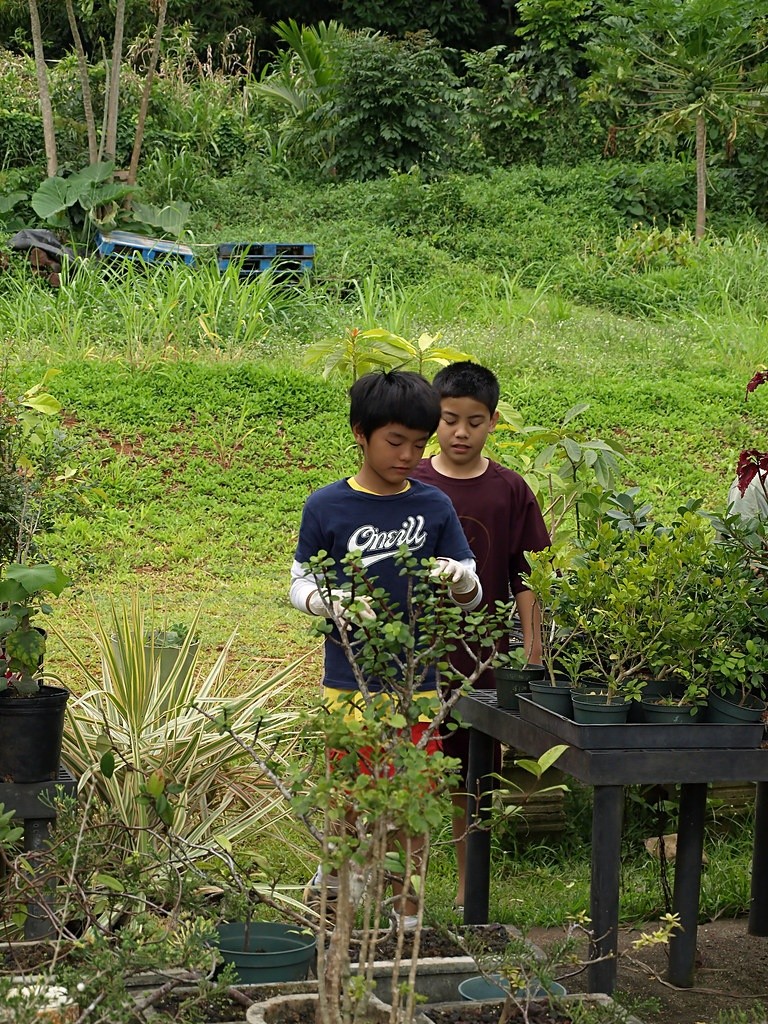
[494,484,768,724]
[110,629,202,727]
[0,563,74,785]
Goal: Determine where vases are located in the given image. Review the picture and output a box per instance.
[201,921,317,986]
[458,972,567,1010]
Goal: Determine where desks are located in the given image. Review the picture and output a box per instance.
[0,759,78,945]
[451,688,768,996]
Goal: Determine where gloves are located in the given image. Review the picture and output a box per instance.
[308,588,377,631]
[421,557,476,594]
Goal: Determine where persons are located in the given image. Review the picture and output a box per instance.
[289,371,484,980]
[727,460,768,577]
[406,360,551,912]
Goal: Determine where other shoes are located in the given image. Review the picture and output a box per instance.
[300,872,338,950]
